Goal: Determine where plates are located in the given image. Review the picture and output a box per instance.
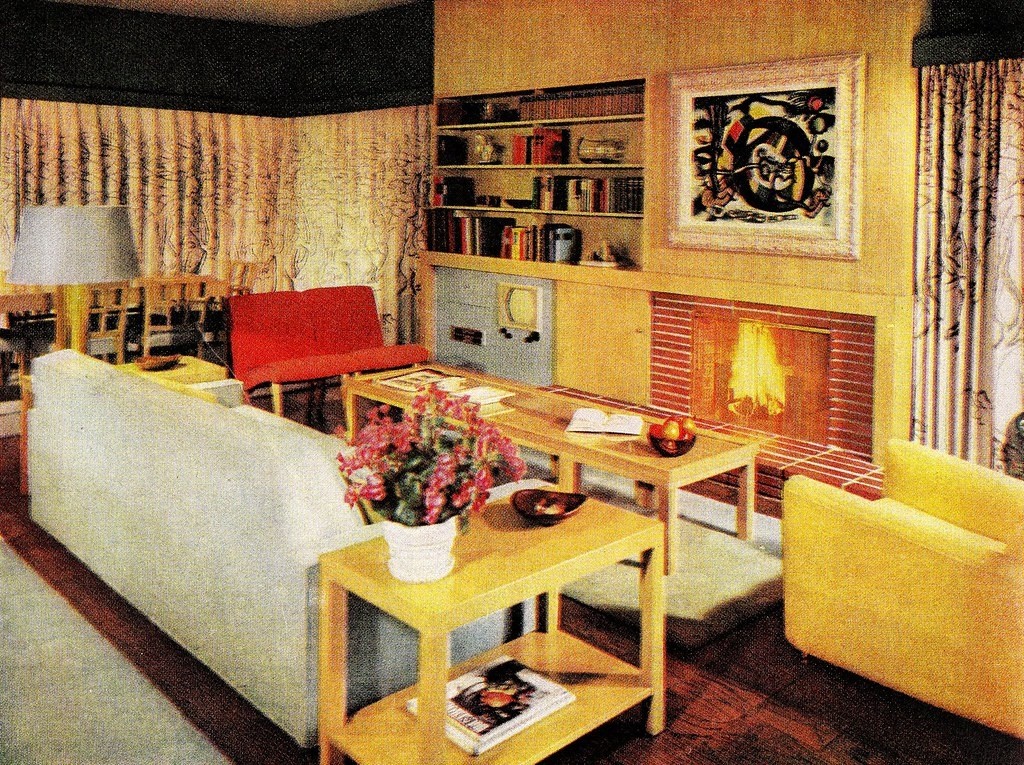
[137,354,180,370]
[511,491,587,523]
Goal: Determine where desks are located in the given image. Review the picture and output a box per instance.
[0,274,221,360]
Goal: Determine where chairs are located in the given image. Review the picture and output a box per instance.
[0,291,63,387]
[55,280,130,363]
[218,257,258,311]
[138,273,215,357]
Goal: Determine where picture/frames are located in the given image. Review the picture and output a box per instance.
[666,52,868,260]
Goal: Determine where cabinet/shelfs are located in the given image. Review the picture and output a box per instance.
[424,73,650,272]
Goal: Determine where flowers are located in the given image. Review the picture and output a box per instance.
[337,384,525,535]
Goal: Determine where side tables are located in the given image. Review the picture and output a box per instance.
[319,486,666,765]
[20,356,227,494]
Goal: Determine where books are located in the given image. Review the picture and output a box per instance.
[427,210,578,262]
[438,101,481,126]
[512,128,564,165]
[405,654,577,756]
[565,408,643,436]
[438,135,468,165]
[519,85,644,121]
[532,176,644,214]
[434,176,476,206]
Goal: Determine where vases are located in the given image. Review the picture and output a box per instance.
[383,518,457,580]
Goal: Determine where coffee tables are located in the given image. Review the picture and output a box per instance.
[342,361,758,575]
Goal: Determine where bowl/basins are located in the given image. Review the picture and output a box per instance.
[647,431,696,457]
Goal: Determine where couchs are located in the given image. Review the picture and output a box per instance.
[782,439,1024,738]
[30,347,536,750]
[223,285,429,418]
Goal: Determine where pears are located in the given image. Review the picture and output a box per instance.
[661,417,697,441]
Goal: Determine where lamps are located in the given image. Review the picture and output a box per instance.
[6,204,141,355]
